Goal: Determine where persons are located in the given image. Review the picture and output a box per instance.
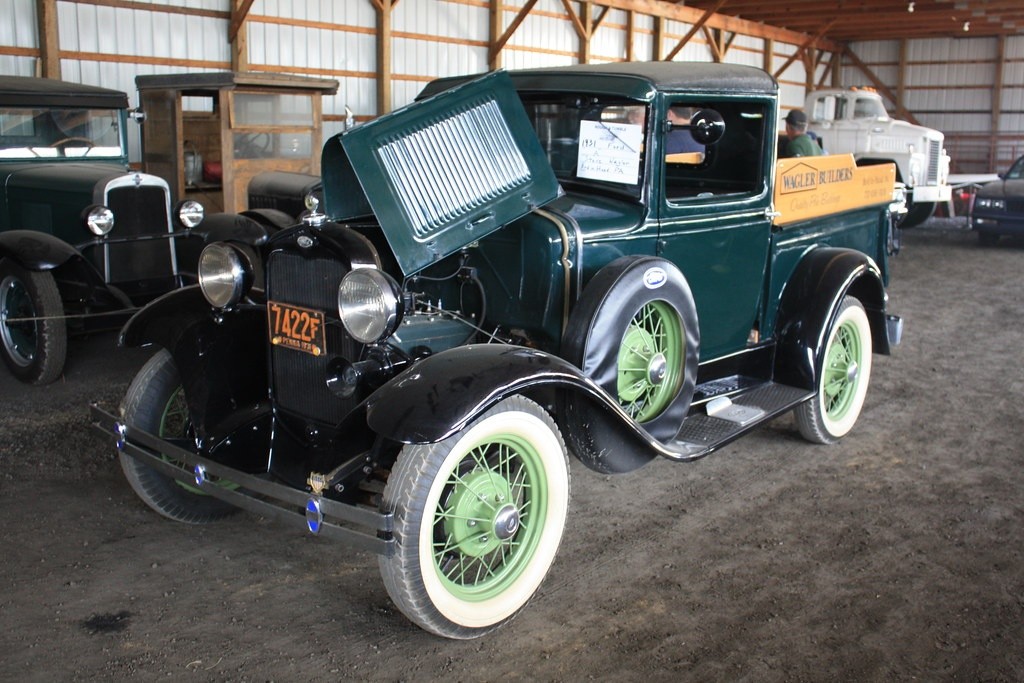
[783,109,827,158]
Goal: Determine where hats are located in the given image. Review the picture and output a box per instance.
[806,131,818,140]
[783,110,807,127]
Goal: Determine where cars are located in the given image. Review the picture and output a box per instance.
[972,155,1024,247]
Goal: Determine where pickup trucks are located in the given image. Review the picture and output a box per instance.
[86,60,902,640]
[115,71,340,232]
[0,72,204,387]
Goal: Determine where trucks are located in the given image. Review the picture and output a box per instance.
[777,86,953,229]
